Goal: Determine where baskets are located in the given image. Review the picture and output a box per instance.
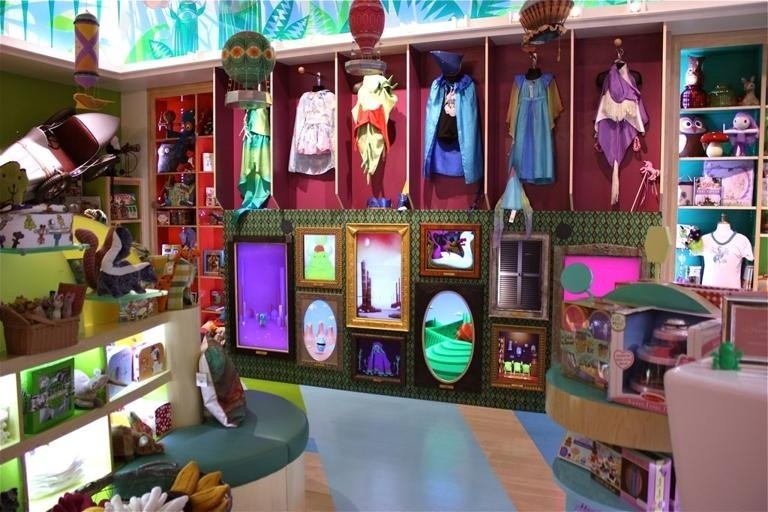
[2,307,79,355]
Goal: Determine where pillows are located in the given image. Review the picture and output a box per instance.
[194,334,248,430]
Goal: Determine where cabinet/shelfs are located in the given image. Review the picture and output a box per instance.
[544,364,676,511]
[148,82,228,343]
[0,301,206,512]
[666,27,767,290]
[80,174,138,242]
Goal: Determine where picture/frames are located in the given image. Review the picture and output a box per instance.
[349,332,408,387]
[343,223,412,335]
[413,280,485,397]
[292,226,344,290]
[294,292,346,373]
[417,221,483,279]
[226,235,295,361]
[488,231,551,321]
[719,295,768,369]
[490,324,547,395]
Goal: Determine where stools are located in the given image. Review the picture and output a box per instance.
[110,387,312,511]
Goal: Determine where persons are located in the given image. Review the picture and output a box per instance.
[692,221,756,290]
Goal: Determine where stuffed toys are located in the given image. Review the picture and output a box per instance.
[680,53,763,207]
[1,95,226,460]
